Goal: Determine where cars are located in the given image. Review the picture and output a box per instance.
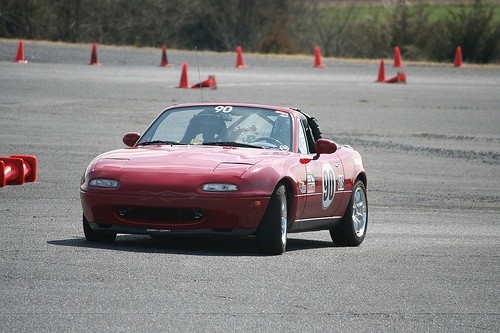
[79,102,372,254]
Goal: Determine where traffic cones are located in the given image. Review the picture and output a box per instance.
[392,45,403,68]
[450,46,463,68]
[14,40,25,63]
[234,45,246,68]
[174,61,190,89]
[191,75,217,90]
[374,59,387,83]
[0,154,36,187]
[88,43,99,67]
[160,44,171,67]
[313,45,325,69]
[383,72,408,84]
